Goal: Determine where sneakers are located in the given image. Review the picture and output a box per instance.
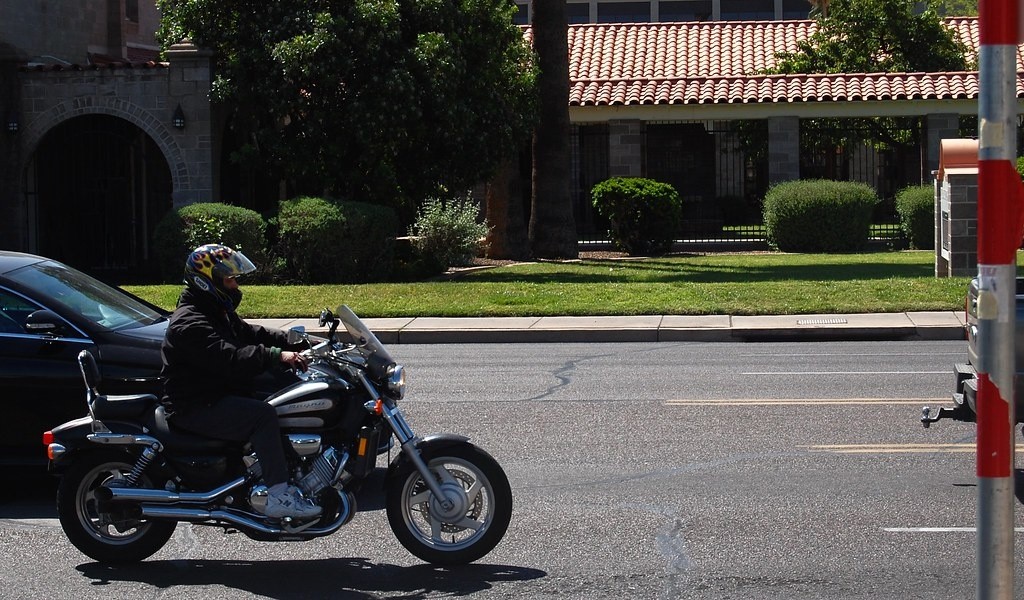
[265,482,322,518]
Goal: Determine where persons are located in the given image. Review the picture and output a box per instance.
[160,243,323,520]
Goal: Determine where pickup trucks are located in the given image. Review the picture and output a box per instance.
[918,274,1024,510]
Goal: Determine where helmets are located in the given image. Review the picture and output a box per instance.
[184,244,257,311]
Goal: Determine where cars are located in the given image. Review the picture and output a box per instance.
[0,250,172,485]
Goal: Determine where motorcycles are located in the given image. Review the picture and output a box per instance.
[41,304,513,568]
